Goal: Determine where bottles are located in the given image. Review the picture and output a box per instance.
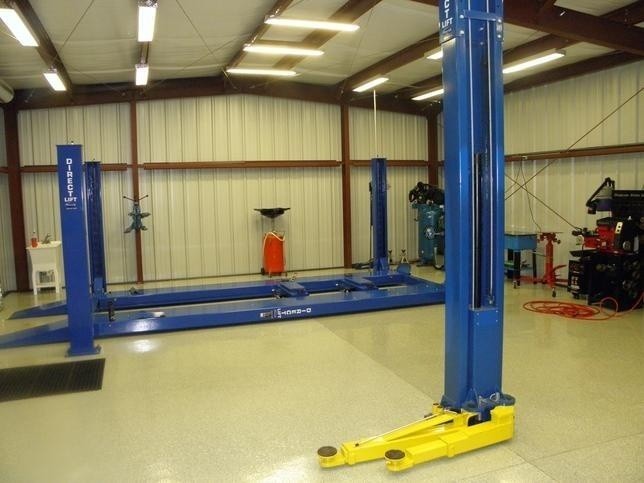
[31,232,38,247]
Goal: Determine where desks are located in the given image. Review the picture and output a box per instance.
[25,241,62,295]
[504,230,538,287]
